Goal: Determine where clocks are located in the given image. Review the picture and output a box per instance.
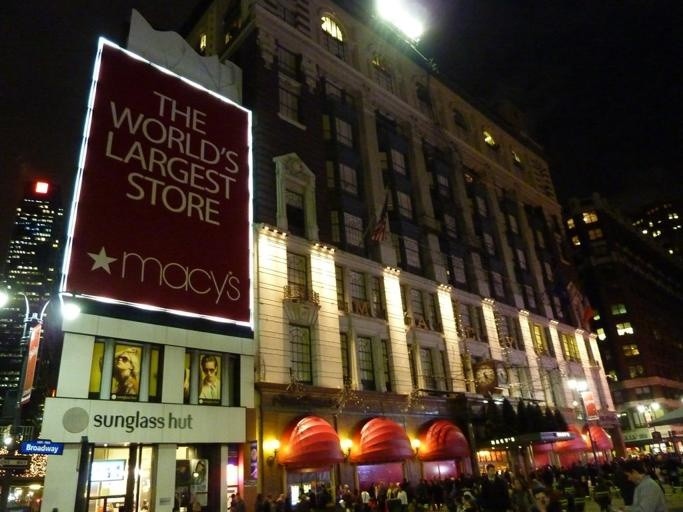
[471,361,497,390]
[495,361,510,388]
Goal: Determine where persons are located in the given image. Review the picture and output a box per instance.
[231,452,683,512]
[99,347,140,395]
[199,353,220,399]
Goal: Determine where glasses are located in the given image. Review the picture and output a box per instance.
[117,356,128,363]
[203,368,217,373]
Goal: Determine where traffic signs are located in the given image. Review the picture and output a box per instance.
[0,455,31,471]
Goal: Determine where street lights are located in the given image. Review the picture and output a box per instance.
[636,401,676,494]
[0,289,79,512]
[567,378,600,466]
